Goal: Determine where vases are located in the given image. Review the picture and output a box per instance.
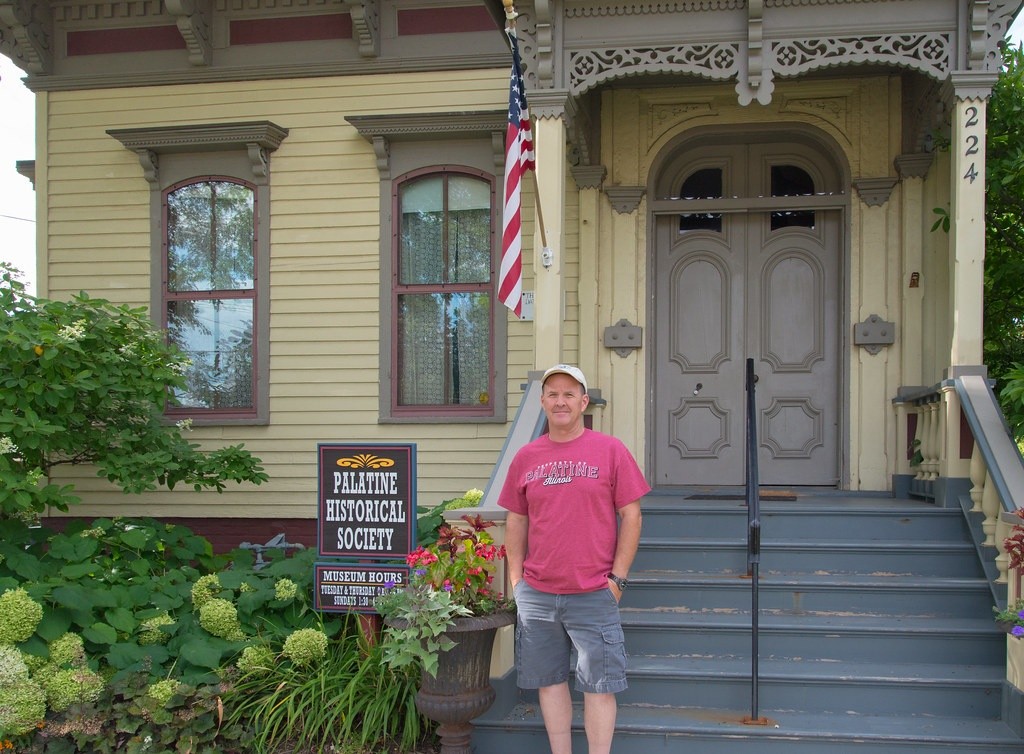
[383,612,516,754]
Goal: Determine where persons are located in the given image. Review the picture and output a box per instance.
[497,364,652,754]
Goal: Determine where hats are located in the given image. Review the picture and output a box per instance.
[540,364,587,393]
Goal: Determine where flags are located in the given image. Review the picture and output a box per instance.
[496,34,536,319]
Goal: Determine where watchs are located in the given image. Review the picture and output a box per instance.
[608,572,627,591]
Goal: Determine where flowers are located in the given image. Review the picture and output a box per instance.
[991,598,1024,639]
[373,488,519,677]
